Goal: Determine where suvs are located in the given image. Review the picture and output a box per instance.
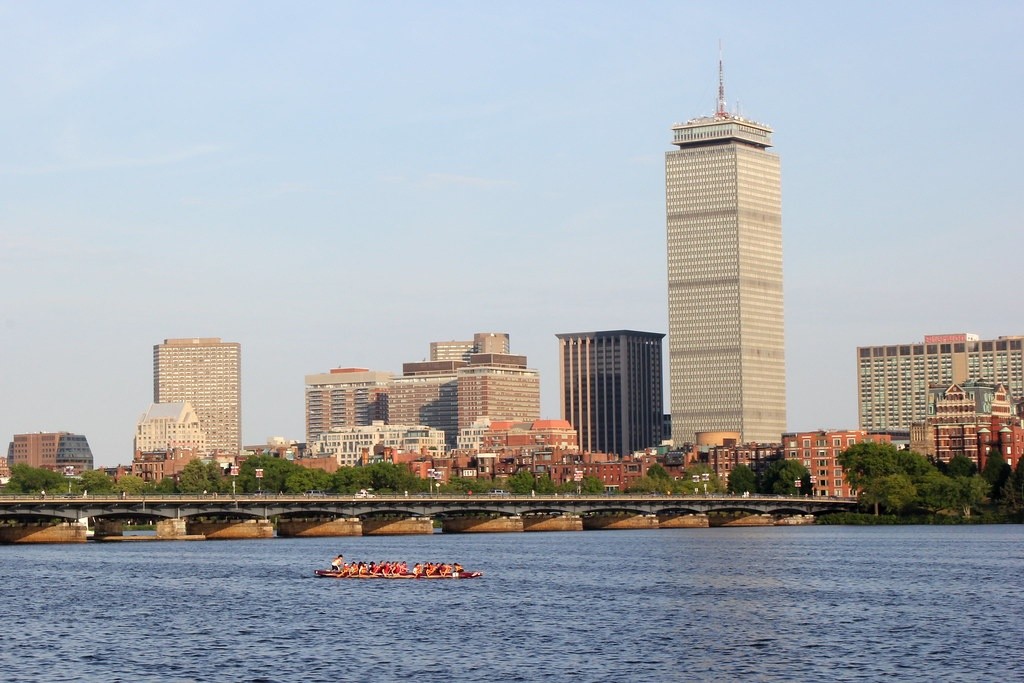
[304,489,327,499]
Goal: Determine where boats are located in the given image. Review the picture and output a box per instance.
[314,568,485,579]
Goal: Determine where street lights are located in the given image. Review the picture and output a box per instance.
[436,482,440,498]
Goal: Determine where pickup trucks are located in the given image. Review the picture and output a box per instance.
[489,489,511,497]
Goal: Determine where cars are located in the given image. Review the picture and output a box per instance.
[564,490,578,497]
[652,491,661,497]
[351,491,377,499]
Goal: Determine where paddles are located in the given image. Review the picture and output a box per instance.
[337,567,452,579]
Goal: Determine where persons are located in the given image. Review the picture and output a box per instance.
[404,490,409,498]
[203,490,208,499]
[341,561,408,575]
[41,490,46,500]
[412,562,464,576]
[743,490,750,498]
[120,488,124,499]
[468,490,472,499]
[667,490,670,497]
[331,554,343,572]
[531,490,535,499]
